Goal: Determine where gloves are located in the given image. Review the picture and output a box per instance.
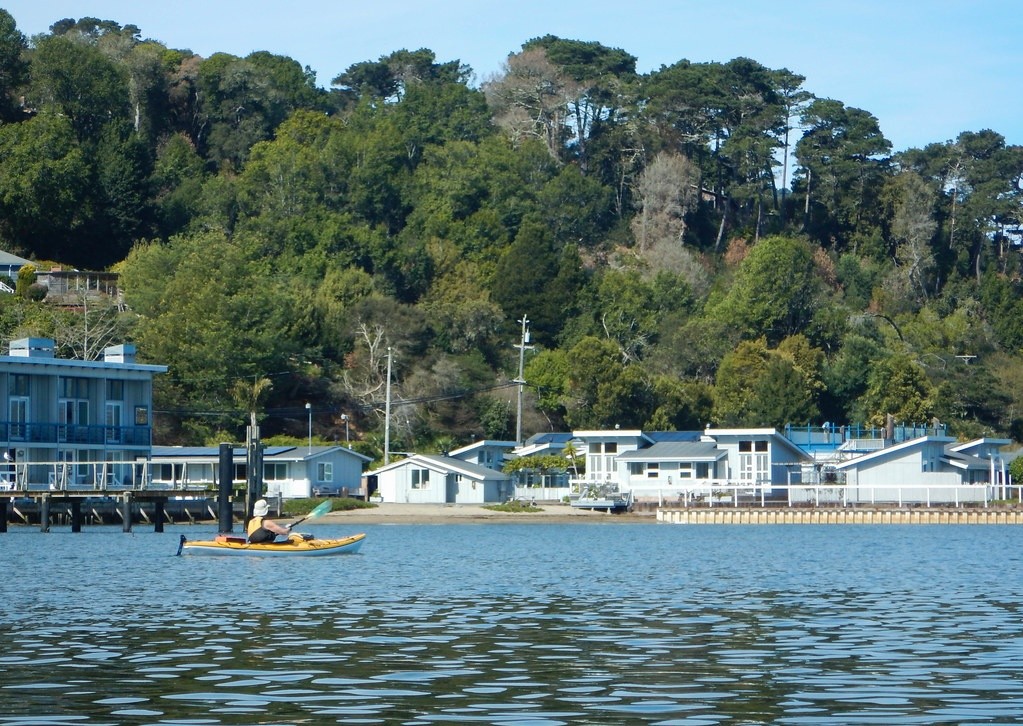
[285,524,292,531]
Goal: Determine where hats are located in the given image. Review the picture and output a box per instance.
[253,499,271,516]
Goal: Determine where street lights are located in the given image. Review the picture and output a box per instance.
[340,414,349,442]
[306,403,311,455]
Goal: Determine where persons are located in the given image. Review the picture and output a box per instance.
[822,420,830,443]
[248,500,291,545]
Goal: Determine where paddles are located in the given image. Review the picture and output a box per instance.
[275,498,333,536]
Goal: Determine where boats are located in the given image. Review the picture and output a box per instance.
[176,533,366,558]
